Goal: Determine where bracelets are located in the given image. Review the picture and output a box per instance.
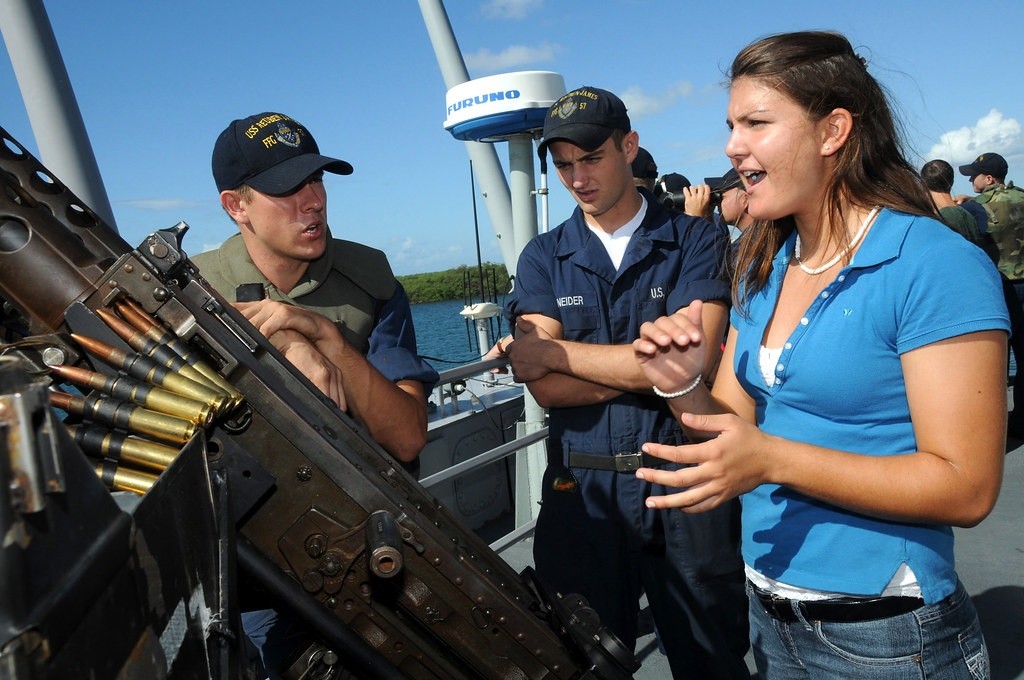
[497,337,506,354]
[652,374,702,399]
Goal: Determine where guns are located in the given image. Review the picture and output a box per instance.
[0,125,643,680]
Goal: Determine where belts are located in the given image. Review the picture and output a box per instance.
[547,449,674,472]
[748,580,925,624]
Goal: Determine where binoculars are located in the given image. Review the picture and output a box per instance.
[673,189,722,209]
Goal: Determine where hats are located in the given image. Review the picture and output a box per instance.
[704,167,742,190]
[632,146,658,179]
[212,112,353,196]
[664,172,691,194]
[959,153,1008,176]
[538,85,631,161]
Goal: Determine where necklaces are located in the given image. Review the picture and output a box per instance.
[794,203,880,274]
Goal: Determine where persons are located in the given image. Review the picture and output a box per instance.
[485,146,758,375]
[187,111,442,680]
[920,152,1024,437]
[632,31,1012,680]
[502,86,749,680]
